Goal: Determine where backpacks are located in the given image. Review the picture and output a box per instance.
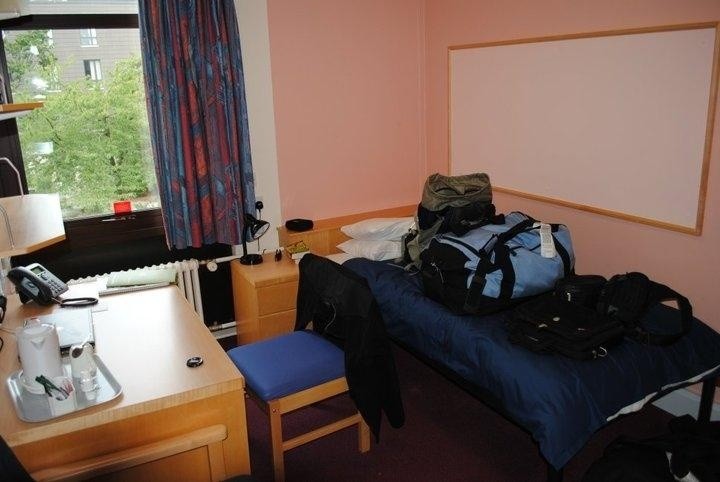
[403,172,495,265]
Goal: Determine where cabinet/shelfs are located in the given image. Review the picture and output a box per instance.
[0,281,251,481]
[1,102,67,259]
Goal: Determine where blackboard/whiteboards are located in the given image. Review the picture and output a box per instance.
[447,21,720,236]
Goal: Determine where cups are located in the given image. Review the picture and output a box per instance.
[68,341,97,380]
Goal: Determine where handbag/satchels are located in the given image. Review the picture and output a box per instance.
[505,272,695,364]
[412,207,577,314]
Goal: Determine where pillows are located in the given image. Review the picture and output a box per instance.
[335,216,418,262]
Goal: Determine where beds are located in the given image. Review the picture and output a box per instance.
[327,250,719,482]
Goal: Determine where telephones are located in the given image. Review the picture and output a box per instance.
[6,262,69,305]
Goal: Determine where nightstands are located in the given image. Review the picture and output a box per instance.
[230,251,301,348]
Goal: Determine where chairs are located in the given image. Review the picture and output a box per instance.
[226,252,373,482]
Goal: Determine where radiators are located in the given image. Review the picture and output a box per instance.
[68,258,205,332]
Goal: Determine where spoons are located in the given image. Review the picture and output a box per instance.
[71,332,92,358]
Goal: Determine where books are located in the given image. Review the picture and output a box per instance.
[96,268,179,293]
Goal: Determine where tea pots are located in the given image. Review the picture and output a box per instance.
[17,317,65,387]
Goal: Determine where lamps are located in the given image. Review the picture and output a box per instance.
[240,213,270,265]
